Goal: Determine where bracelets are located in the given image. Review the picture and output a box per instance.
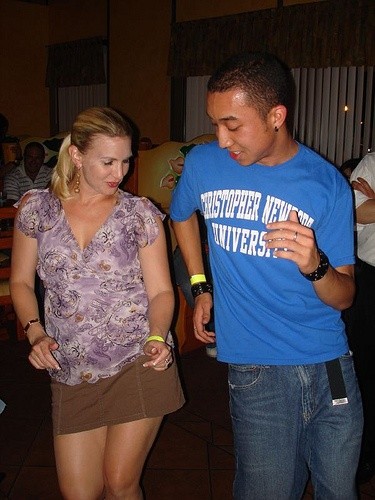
[24,319,38,335]
[190,274,206,285]
[191,282,213,299]
[146,336,164,342]
[299,250,329,281]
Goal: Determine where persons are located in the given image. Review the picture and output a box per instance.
[169,54,364,500]
[10,107,187,500]
[338,152,375,485]
[162,143,217,356]
[0,114,54,208]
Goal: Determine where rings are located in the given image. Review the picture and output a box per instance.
[295,232,297,240]
[166,359,169,363]
